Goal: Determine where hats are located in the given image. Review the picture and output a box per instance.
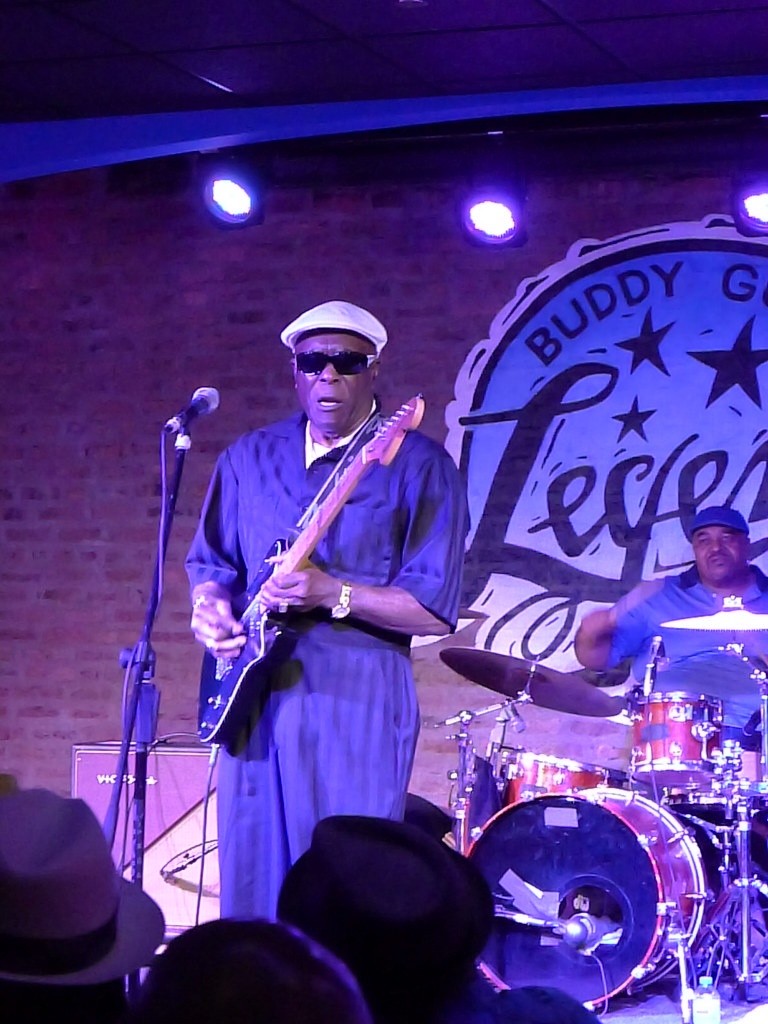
[687,506,749,539]
[276,812,496,1008]
[280,300,388,357]
[0,789,166,986]
[141,918,373,1024]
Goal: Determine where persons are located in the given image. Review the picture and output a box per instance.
[574,506,768,735]
[276,815,495,1024]
[130,915,373,1024]
[183,300,470,924]
[0,788,164,1024]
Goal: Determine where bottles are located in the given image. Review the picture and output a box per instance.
[693,976,720,1024]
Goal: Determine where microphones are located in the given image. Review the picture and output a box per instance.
[743,710,762,736]
[649,637,669,672]
[503,699,525,734]
[164,388,220,434]
[512,912,598,950]
[159,869,177,885]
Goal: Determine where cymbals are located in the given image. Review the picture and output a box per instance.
[437,646,622,718]
[659,611,768,630]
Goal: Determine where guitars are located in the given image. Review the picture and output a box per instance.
[198,394,426,745]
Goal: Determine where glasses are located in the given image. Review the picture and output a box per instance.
[295,351,376,376]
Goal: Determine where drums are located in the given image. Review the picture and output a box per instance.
[464,788,707,1006]
[508,752,627,805]
[630,691,768,812]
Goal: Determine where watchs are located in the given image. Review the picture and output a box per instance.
[332,581,352,620]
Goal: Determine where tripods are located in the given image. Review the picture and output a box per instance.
[674,796,768,1004]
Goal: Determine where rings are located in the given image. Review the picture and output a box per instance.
[279,603,288,613]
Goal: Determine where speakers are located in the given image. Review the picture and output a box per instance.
[68,741,223,941]
